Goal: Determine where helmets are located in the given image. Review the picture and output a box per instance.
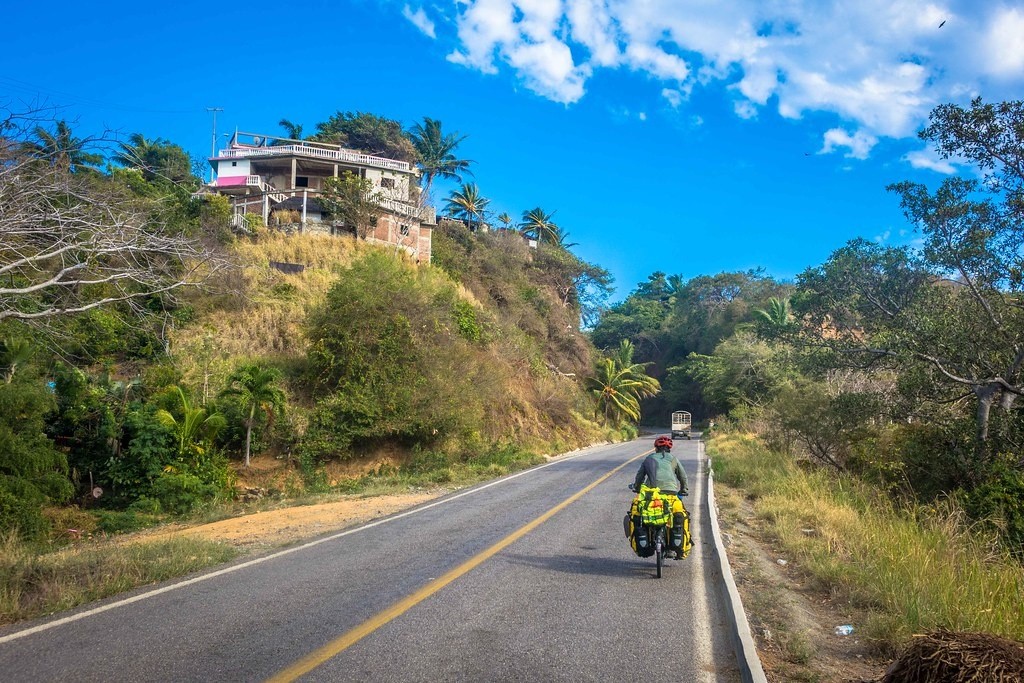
[653,436,673,448]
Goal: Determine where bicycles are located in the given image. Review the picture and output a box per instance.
[631,490,689,578]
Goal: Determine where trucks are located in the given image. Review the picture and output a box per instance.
[671,410,691,440]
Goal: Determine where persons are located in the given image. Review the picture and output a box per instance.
[633,436,689,558]
